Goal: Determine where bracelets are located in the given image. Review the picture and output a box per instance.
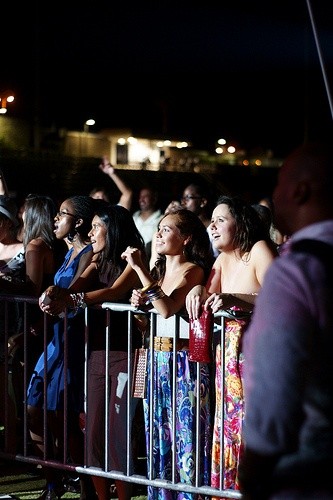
[140,281,165,301]
[71,293,88,310]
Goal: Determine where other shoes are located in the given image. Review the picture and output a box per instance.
[68,481,81,493]
[35,484,68,500]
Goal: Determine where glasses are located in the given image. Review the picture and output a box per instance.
[182,195,199,202]
[59,209,76,217]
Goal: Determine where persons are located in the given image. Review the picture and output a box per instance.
[185,195,276,500]
[26,195,111,499]
[119,208,215,499]
[239,144,333,500]
[38,206,150,499]
[87,154,132,211]
[132,183,290,250]
[0,197,60,458]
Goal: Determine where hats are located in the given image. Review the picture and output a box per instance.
[0,195,19,226]
